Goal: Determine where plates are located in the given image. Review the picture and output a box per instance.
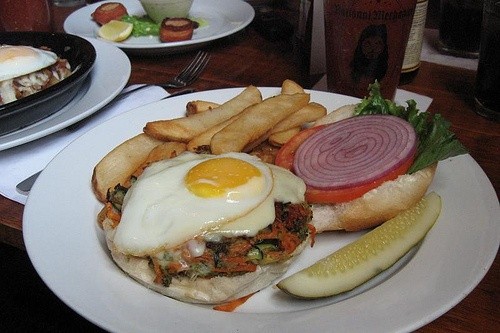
[0,36,131,153]
[22,86,500,333]
[63,1,256,55]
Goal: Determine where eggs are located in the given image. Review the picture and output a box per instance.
[108,151,306,257]
[0,45,57,82]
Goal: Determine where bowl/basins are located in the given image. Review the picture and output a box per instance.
[1,31,97,136]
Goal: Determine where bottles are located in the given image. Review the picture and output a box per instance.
[398,0,428,86]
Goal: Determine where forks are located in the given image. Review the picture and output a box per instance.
[66,51,212,132]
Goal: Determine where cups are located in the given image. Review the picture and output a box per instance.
[472,0,500,121]
[436,0,480,60]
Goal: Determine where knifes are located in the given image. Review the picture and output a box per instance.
[17,88,200,195]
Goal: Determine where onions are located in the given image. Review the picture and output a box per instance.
[294,114,417,188]
